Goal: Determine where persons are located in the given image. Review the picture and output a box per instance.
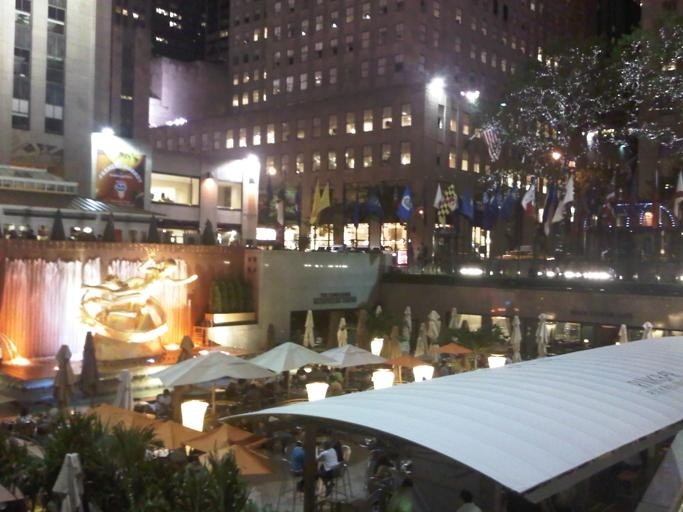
[416,242,456,275]
[39,404,58,432]
[287,423,415,509]
[20,408,38,433]
[457,489,482,511]
[158,193,172,202]
[7,220,48,241]
[70,227,80,240]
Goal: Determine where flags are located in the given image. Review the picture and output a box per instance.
[481,124,502,163]
[262,170,683,238]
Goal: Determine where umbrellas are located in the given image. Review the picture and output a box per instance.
[203,219,217,245]
[147,213,159,243]
[52,452,86,512]
[103,211,115,243]
[51,208,64,240]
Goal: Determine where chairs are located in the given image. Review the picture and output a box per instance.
[274,444,355,511]
